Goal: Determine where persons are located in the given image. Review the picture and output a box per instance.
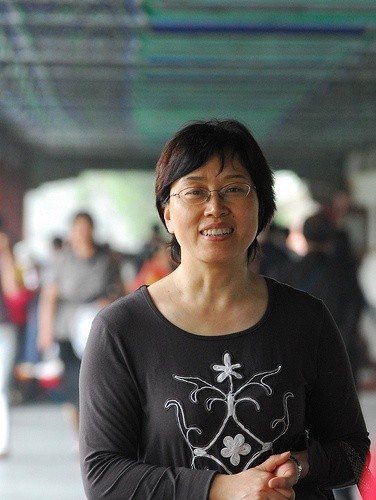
[75,114,373,500]
[0,168,376,462]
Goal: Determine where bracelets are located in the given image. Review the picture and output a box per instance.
[289,455,303,486]
